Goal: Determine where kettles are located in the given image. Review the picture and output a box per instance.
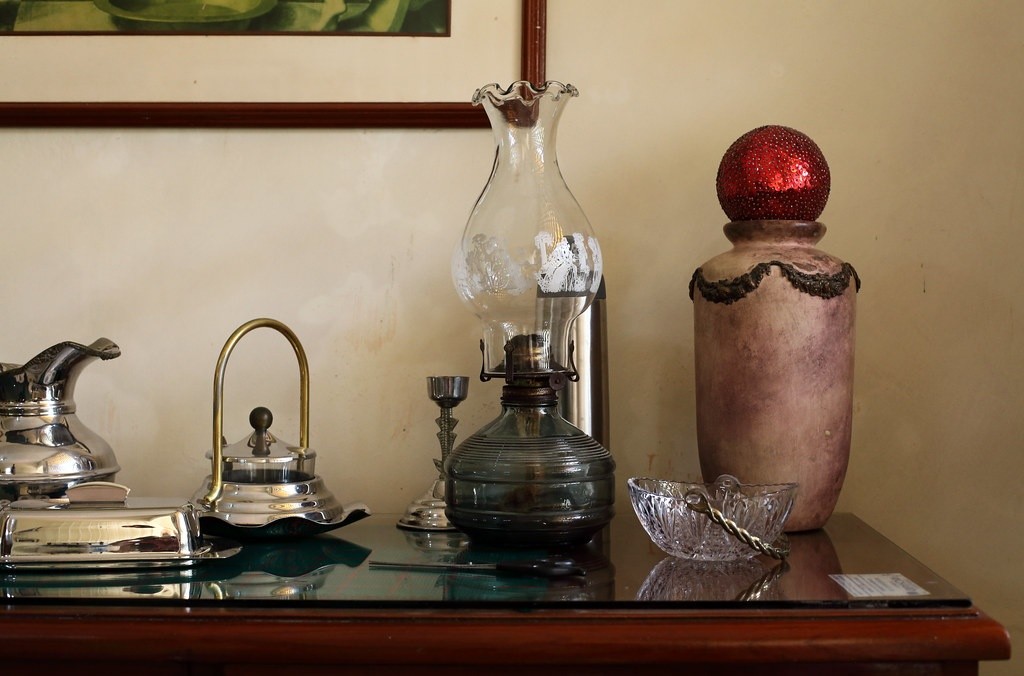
[191,318,343,525]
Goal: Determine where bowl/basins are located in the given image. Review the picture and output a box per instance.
[628,473,800,562]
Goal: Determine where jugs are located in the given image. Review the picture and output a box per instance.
[0,337,121,502]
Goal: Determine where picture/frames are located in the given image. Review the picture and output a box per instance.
[0,0,547,129]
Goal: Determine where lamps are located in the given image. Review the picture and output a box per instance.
[445,80,617,552]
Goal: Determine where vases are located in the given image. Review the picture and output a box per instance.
[694,528,848,602]
[689,218,861,533]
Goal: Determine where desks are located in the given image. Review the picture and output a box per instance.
[0,508,1015,676]
